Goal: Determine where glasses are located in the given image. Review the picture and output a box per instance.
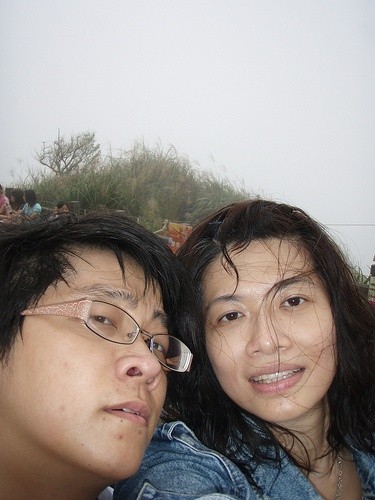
[20,300,194,373]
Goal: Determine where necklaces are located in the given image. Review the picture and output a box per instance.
[335,449,344,500]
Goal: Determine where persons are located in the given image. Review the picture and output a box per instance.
[5,189,42,223]
[0,213,195,500]
[0,183,70,223]
[114,199,374,499]
